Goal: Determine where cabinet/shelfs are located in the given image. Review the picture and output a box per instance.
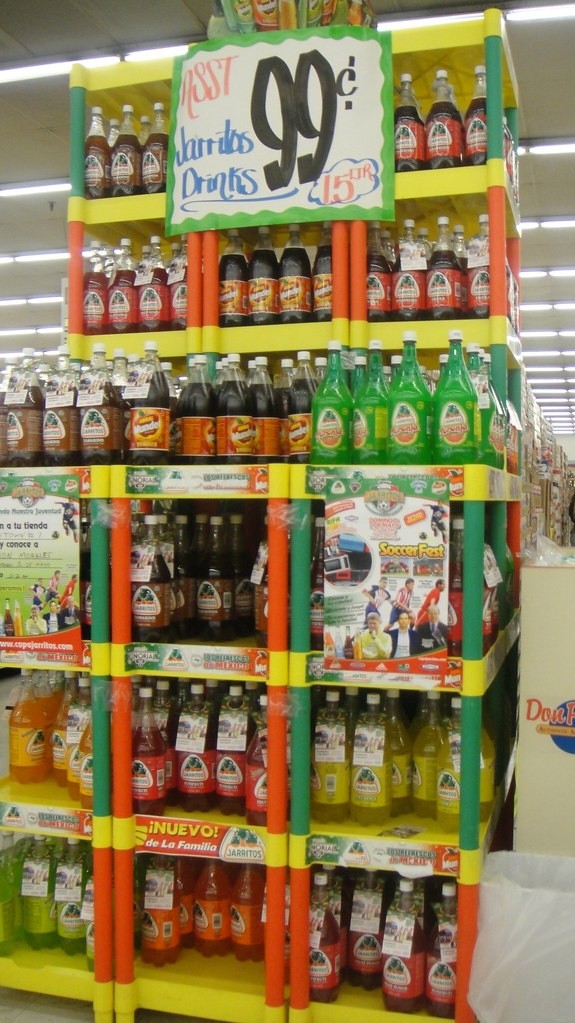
[0,6,526,1023]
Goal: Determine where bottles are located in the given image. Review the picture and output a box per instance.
[5,598,23,638]
[308,863,457,1019]
[284,872,291,986]
[483,545,498,654]
[479,725,495,823]
[446,514,464,654]
[323,624,354,660]
[0,828,95,972]
[367,213,520,339]
[79,501,91,640]
[0,329,518,474]
[82,102,170,198]
[82,234,187,334]
[504,542,514,628]
[394,65,520,205]
[10,667,92,810]
[311,515,323,651]
[133,852,266,968]
[311,685,461,834]
[219,220,334,326]
[133,514,268,643]
[285,695,292,819]
[130,674,269,826]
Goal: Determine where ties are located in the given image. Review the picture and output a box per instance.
[69,608,72,616]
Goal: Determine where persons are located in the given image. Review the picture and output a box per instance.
[59,574,78,610]
[383,578,416,631]
[362,577,394,630]
[568,494,575,546]
[40,570,62,609]
[56,497,80,543]
[59,596,79,629]
[31,578,46,611]
[416,605,447,654]
[43,601,65,634]
[413,579,446,628]
[26,605,48,635]
[385,610,422,659]
[360,612,393,660]
[424,498,448,544]
